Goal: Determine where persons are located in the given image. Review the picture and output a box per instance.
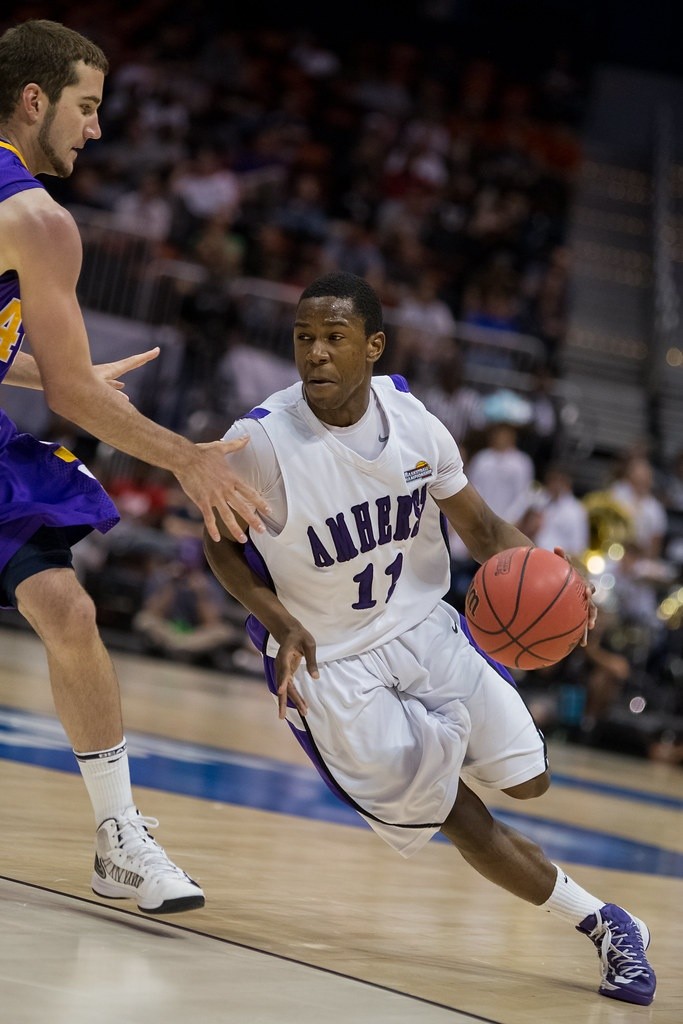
[201,274,657,1007]
[25,41,682,749]
[0,17,271,914]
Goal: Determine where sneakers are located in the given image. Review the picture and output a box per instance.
[91,805,206,915]
[575,902,657,1006]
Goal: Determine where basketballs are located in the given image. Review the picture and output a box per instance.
[464,546,588,670]
